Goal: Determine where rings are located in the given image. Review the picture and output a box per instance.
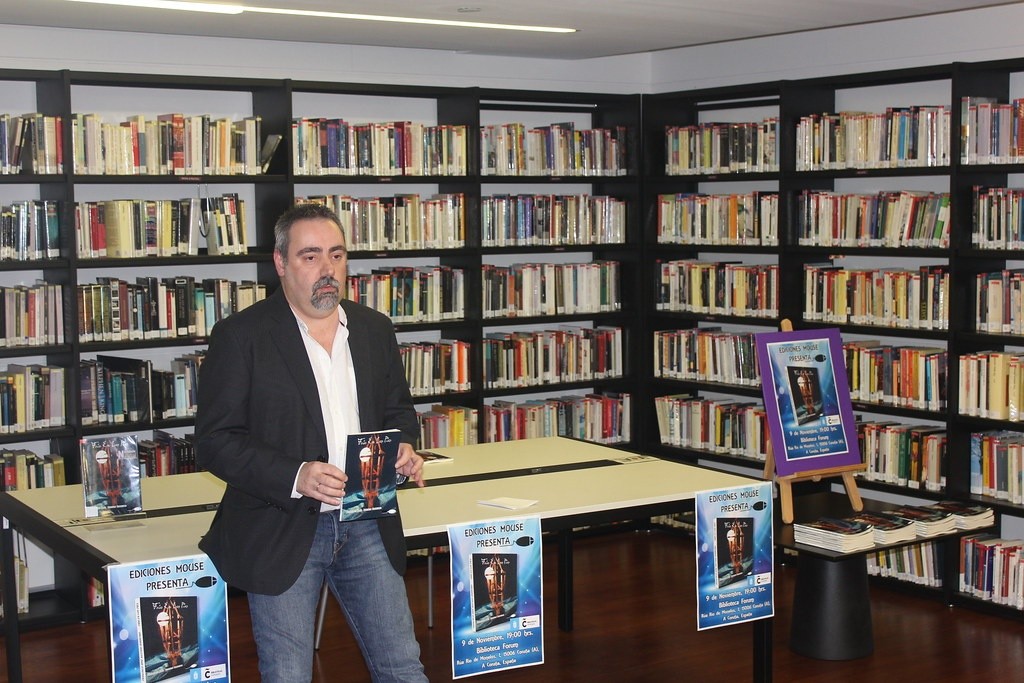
[316,483,321,491]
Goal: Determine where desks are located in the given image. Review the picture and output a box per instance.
[1,437,771,683]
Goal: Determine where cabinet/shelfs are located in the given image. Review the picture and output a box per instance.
[1,56,1024,626]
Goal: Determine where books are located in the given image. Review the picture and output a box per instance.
[656,97,1024,611]
[416,450,453,463]
[0,111,630,609]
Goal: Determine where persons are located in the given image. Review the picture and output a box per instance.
[192,204,430,683]
